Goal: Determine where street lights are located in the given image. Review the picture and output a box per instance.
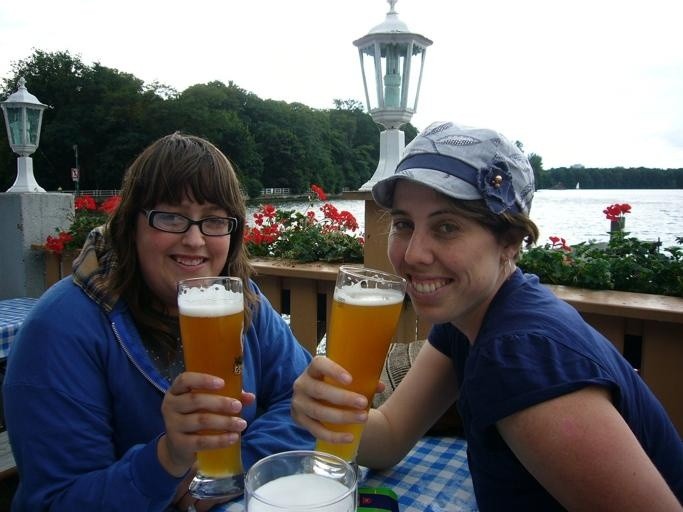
[72,145,79,193]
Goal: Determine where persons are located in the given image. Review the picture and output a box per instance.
[290,119,683,512]
[0,133,308,512]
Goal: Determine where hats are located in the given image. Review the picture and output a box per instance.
[371,120,535,219]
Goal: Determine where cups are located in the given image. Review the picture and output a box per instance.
[314,266,407,480]
[244,449,359,511]
[179,277,245,481]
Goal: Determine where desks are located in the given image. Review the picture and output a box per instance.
[0,296,41,361]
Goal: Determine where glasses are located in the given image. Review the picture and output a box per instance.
[138,206,238,237]
